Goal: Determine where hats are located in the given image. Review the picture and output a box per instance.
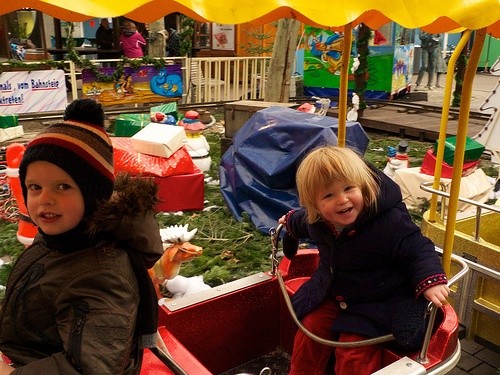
[18,98,115,215]
[98,18,112,24]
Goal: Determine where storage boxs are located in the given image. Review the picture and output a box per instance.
[221,101,301,155]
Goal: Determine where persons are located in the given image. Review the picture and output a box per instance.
[0,120,152,375]
[95,19,146,58]
[415,30,448,90]
[276,145,452,375]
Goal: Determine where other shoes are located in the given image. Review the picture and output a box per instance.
[414,85,419,91]
[424,84,434,90]
[435,84,444,88]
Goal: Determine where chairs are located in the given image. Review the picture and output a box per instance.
[278,250,459,370]
